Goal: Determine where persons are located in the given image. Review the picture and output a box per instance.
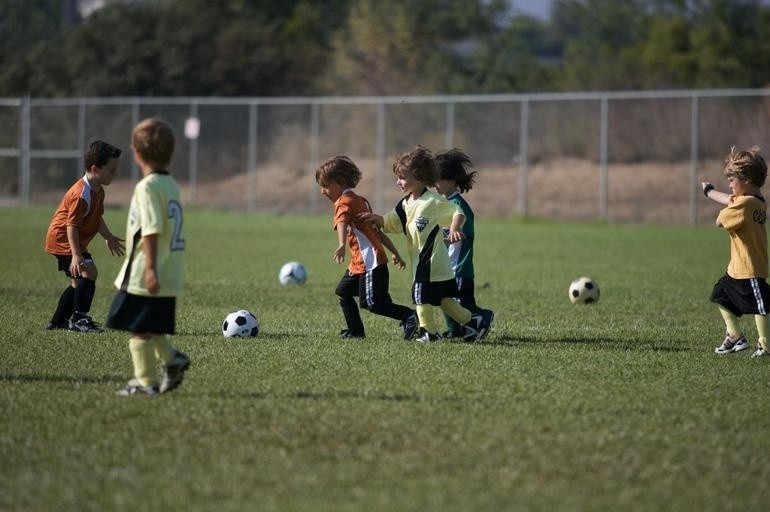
[431,148,494,341]
[355,144,484,345]
[315,156,419,342]
[104,117,191,397]
[44,140,126,334]
[702,145,770,358]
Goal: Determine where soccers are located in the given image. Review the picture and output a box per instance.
[221,309,260,339]
[569,276,600,304]
[278,262,307,286]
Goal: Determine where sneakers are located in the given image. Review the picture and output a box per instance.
[114,378,159,396]
[340,328,366,340]
[47,319,69,329]
[714,329,749,354]
[751,343,770,358]
[159,351,190,394]
[65,311,104,333]
[404,307,494,343]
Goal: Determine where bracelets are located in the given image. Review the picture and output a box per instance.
[704,184,715,197]
